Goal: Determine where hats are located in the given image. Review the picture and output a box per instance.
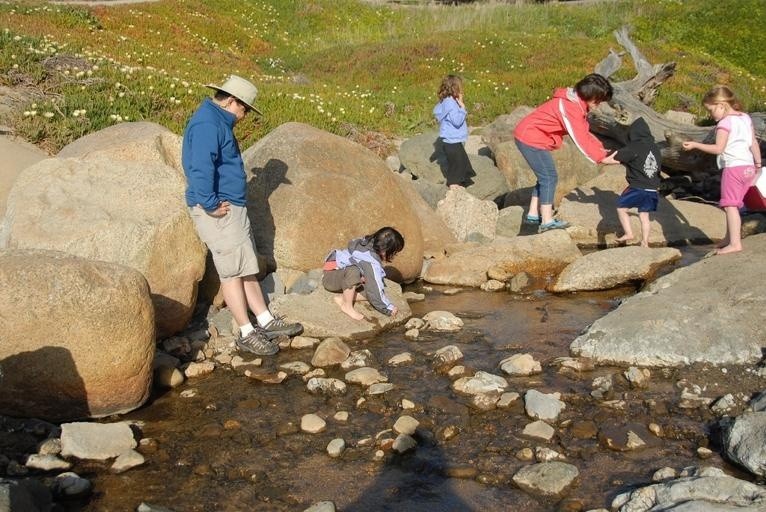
[205,75,263,116]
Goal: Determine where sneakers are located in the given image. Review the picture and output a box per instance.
[257,316,303,340]
[237,329,280,356]
[526,216,570,234]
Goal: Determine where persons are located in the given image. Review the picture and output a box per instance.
[682,86,762,256]
[432,75,476,190]
[512,73,621,234]
[182,74,304,356]
[322,227,405,320]
[604,116,663,248]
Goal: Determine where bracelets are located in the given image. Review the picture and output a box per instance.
[754,162,762,165]
